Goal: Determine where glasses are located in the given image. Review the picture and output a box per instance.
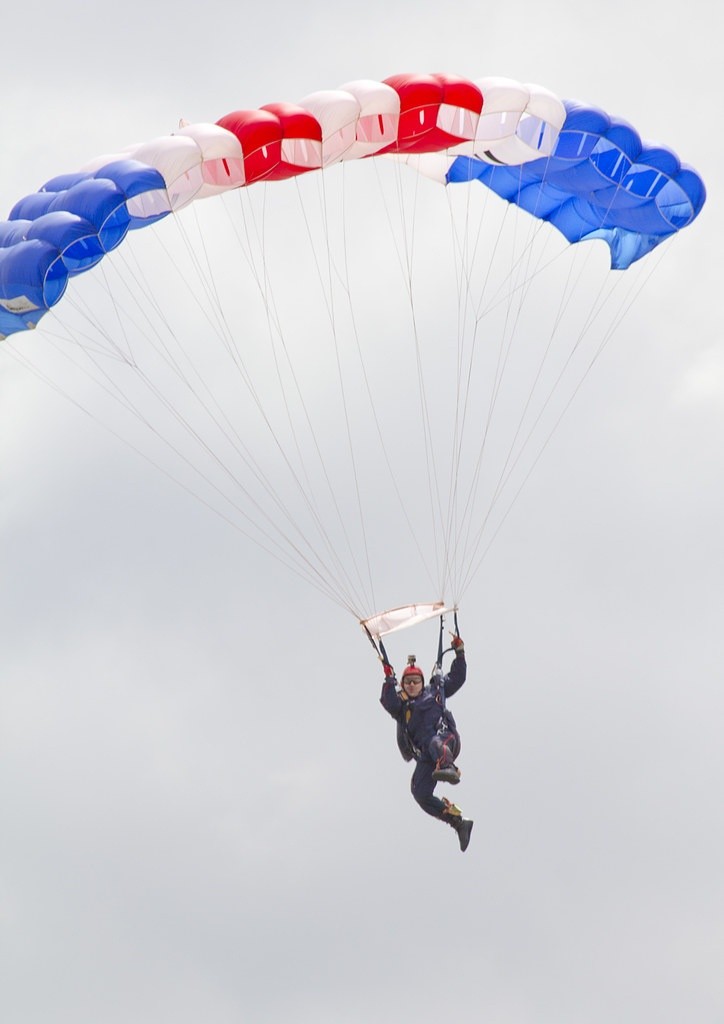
[404,677,423,685]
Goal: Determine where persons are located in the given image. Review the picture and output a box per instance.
[378,638,475,852]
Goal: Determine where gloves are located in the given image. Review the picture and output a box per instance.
[451,636,463,653]
[384,665,394,679]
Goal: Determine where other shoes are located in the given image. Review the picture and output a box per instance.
[431,767,460,785]
[456,817,473,851]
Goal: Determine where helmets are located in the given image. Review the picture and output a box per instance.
[402,667,425,687]
